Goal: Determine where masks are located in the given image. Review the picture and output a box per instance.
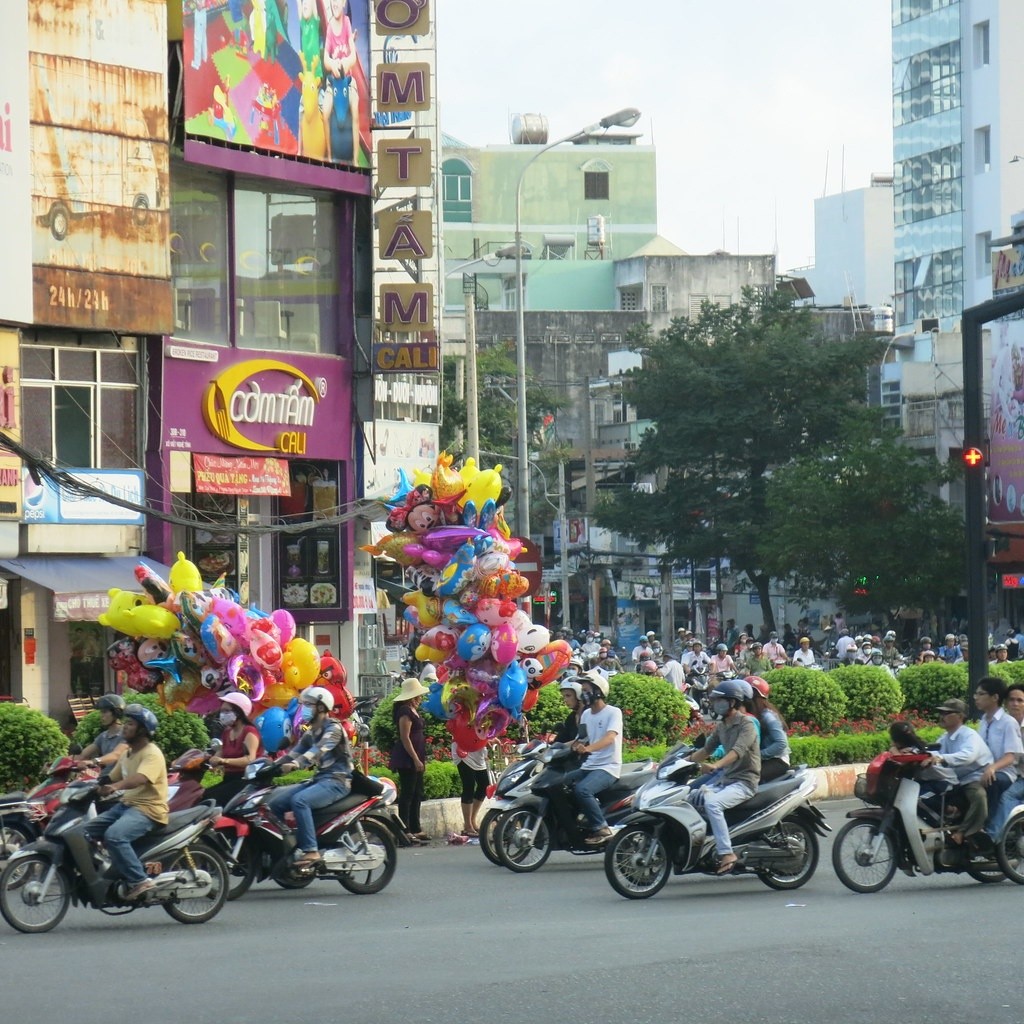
[219,712,237,727]
[713,701,731,715]
[580,691,596,706]
[300,705,318,723]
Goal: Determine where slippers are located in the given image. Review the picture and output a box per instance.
[293,857,321,872]
[461,829,480,837]
[716,859,737,876]
[584,828,614,845]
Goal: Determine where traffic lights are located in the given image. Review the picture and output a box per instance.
[962,447,984,468]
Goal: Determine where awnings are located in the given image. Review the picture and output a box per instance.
[0,551,214,622]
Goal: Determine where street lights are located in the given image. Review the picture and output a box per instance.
[514,105,643,622]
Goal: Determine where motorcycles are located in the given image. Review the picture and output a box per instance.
[688,659,741,720]
[604,734,833,900]
[831,743,1024,893]
[474,713,663,873]
[0,725,416,935]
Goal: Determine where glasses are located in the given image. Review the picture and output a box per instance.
[939,710,960,715]
[973,691,991,696]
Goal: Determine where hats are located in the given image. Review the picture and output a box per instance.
[393,677,430,701]
[936,698,969,717]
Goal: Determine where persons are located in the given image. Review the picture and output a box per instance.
[915,608,932,638]
[192,692,267,838]
[90,629,104,696]
[913,623,1024,665]
[387,677,433,843]
[47,694,130,816]
[724,619,792,676]
[930,609,967,638]
[632,627,738,691]
[549,626,624,674]
[563,675,622,844]
[683,676,792,876]
[269,687,356,869]
[792,612,905,677]
[96,704,169,898]
[452,735,490,836]
[71,628,88,698]
[550,675,586,743]
[901,677,1024,853]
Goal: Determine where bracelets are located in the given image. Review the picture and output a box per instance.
[93,758,101,764]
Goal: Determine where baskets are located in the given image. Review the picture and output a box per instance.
[854,773,887,805]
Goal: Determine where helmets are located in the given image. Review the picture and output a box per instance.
[710,676,769,699]
[579,671,610,698]
[93,687,334,738]
[555,626,1016,671]
[559,676,582,701]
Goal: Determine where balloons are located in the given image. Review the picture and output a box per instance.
[99,551,321,720]
[313,648,356,737]
[415,609,573,757]
[294,704,318,738]
[255,696,300,752]
[358,449,529,630]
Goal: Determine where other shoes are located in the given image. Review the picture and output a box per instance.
[407,834,419,842]
[418,831,432,839]
[126,884,154,900]
[946,826,995,846]
